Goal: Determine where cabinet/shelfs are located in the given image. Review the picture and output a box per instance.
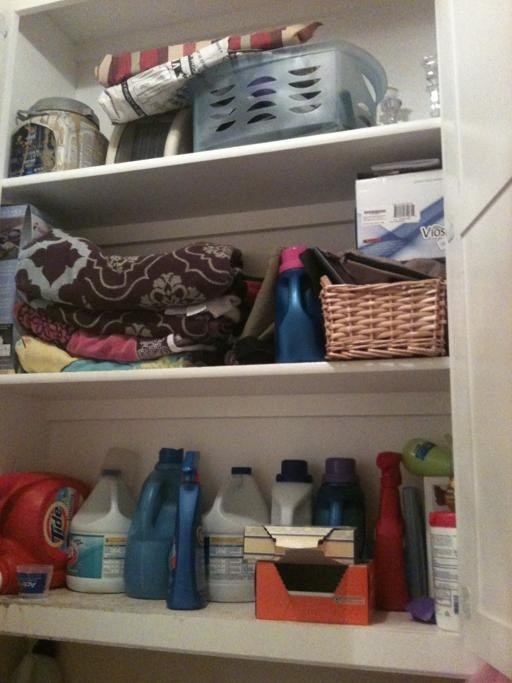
[0,2,510,677]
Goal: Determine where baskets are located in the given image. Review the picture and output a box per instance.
[319,276,447,360]
